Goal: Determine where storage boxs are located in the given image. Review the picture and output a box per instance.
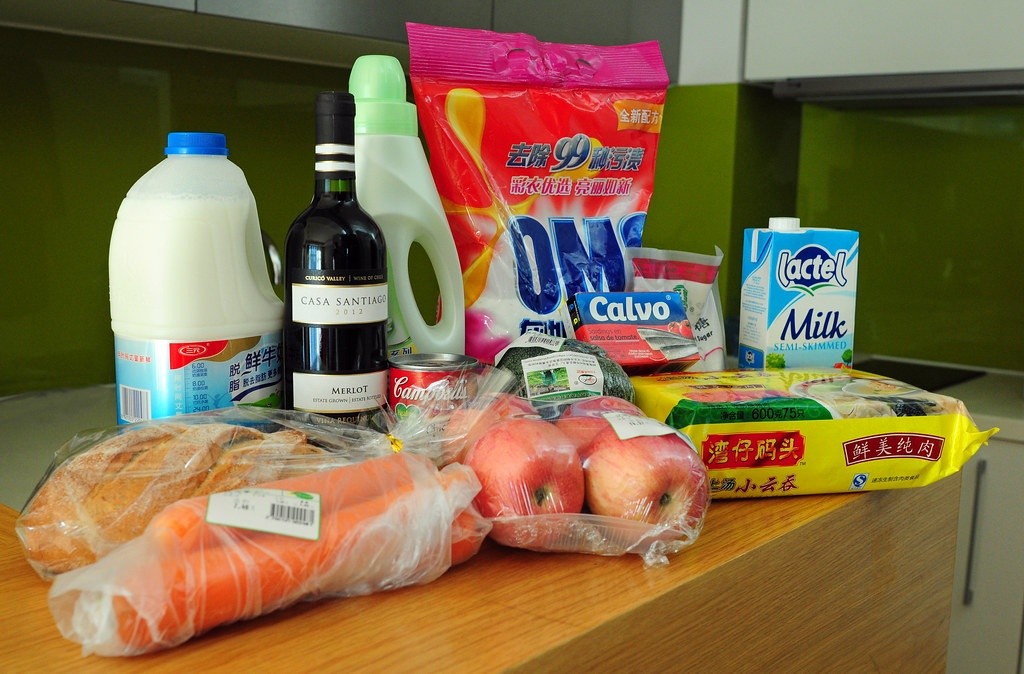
[560,291,700,375]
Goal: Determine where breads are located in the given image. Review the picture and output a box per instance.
[19,424,341,582]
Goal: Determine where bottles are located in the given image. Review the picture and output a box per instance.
[108,131,287,433]
[347,54,465,361]
[286,89,388,453]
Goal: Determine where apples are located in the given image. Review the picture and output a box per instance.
[443,396,709,553]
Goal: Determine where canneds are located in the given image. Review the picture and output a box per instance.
[386,354,480,432]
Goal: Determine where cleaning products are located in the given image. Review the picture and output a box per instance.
[347,54,465,360]
[404,20,671,367]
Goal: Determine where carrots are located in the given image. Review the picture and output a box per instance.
[107,453,482,646]
